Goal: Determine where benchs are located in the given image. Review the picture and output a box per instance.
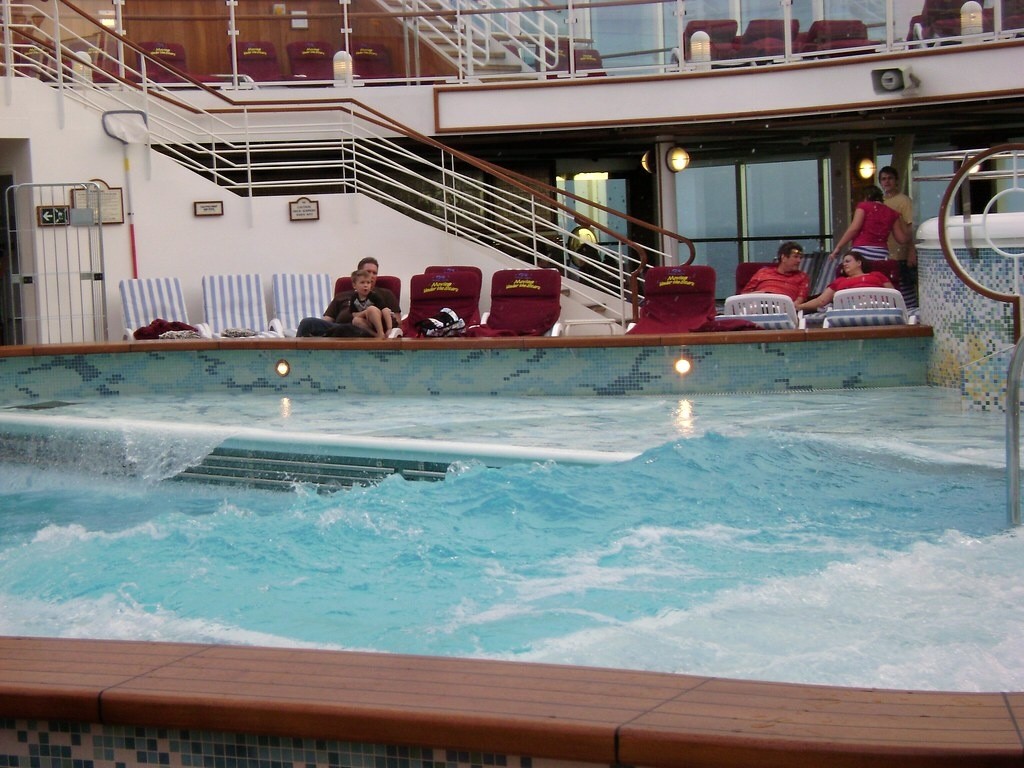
[905,1,1024,38]
[136,41,225,91]
[669,18,875,64]
[227,39,435,86]
[535,37,607,83]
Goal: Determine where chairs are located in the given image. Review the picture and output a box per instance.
[119,278,208,340]
[202,274,284,339]
[273,274,334,342]
[386,266,482,339]
[467,268,562,339]
[823,286,920,328]
[804,252,844,322]
[625,265,717,334]
[714,262,807,330]
[309,276,400,329]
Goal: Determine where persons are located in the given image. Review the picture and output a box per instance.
[879,166,918,309]
[742,242,810,308]
[527,236,625,294]
[349,270,393,340]
[296,257,402,338]
[797,252,896,310]
[828,185,911,261]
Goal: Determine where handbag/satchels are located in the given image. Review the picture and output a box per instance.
[414,307,465,339]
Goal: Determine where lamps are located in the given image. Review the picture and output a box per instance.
[689,30,711,73]
[72,52,92,90]
[641,149,656,173]
[665,145,689,173]
[960,2,983,35]
[853,156,876,180]
[332,50,351,80]
